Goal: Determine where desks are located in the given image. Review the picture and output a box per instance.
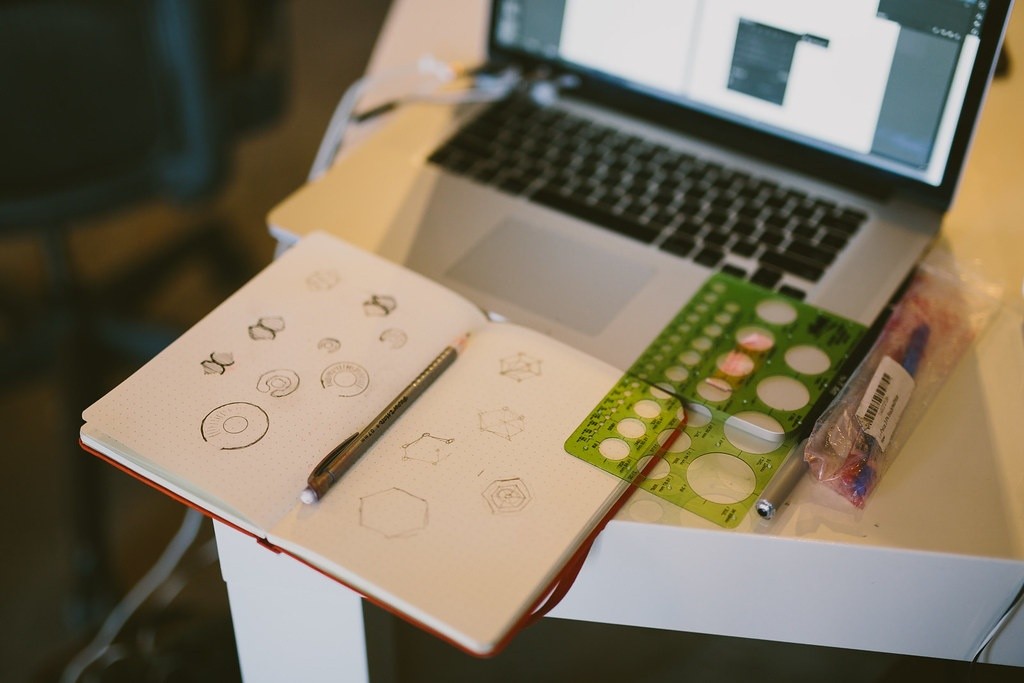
[208,1,1023,683]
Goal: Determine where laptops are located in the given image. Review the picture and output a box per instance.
[264,0,1014,439]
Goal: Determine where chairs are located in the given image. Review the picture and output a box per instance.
[1,2,293,642]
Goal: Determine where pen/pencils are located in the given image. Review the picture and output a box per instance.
[851,323,931,499]
[753,347,891,520]
[299,330,471,505]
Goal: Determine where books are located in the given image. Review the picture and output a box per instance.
[77,231,685,656]
